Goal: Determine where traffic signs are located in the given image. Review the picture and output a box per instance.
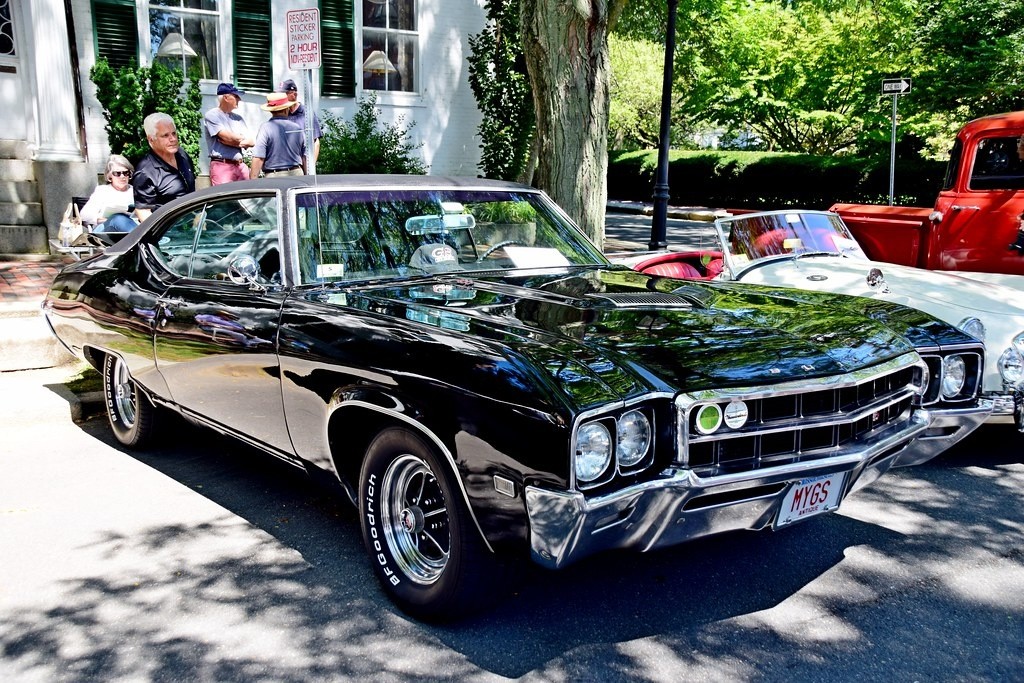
[882,77,912,95]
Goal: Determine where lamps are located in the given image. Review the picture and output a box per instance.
[363,34,396,74]
[158,28,197,59]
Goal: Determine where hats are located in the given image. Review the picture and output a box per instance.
[275,79,297,93]
[217,83,245,96]
[260,92,297,111]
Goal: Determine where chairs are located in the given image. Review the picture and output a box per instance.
[646,262,699,284]
[704,259,726,277]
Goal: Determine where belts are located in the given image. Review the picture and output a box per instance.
[211,157,243,164]
[265,164,301,173]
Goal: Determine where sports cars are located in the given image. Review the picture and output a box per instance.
[602,209,1023,437]
[40,173,997,631]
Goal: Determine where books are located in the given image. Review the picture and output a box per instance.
[103,205,135,218]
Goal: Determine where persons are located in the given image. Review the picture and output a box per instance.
[250,92,306,229]
[1016,134,1024,231]
[204,83,255,187]
[282,79,323,175]
[79,154,139,232]
[133,112,197,233]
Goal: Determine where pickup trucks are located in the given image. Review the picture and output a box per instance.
[826,110,1024,273]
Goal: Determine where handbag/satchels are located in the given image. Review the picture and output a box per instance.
[58,203,84,245]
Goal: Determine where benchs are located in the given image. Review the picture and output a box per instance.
[49,196,132,259]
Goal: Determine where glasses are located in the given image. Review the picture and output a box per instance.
[109,170,130,176]
